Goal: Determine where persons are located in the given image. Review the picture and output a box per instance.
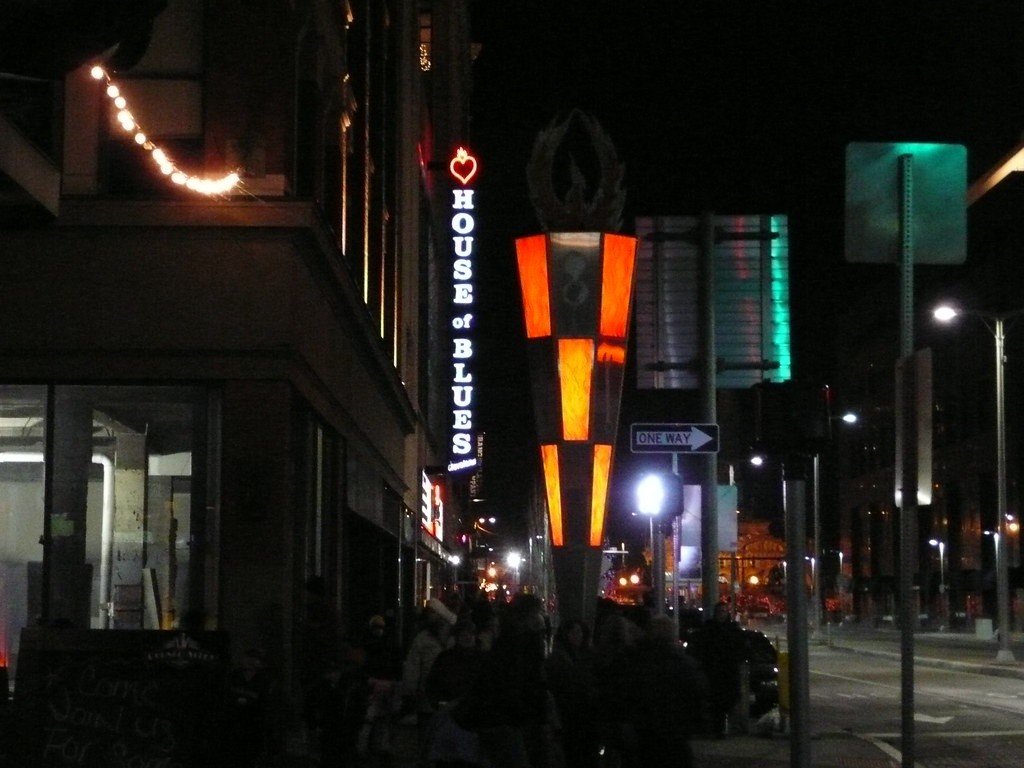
[327,592,776,767]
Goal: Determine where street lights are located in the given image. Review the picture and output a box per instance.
[930,538,944,635]
[931,306,1016,665]
[814,412,859,646]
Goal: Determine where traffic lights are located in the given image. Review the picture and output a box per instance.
[801,380,831,450]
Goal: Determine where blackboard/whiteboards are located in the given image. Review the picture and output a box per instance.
[4,626,232,768]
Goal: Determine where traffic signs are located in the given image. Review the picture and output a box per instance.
[629,422,721,455]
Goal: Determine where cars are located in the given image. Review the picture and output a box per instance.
[742,629,781,715]
